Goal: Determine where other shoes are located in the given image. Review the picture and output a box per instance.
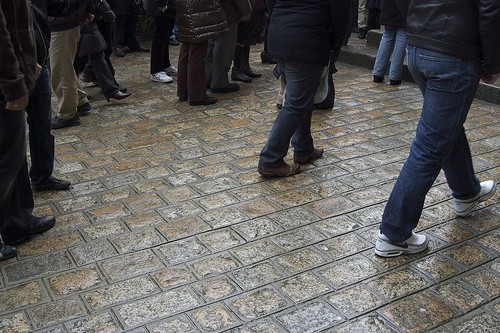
[389,79,402,85]
[374,75,384,83]
[258,162,300,177]
[293,148,323,164]
[358,29,366,38]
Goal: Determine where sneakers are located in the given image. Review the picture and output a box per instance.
[3,246,16,261]
[454,180,496,217]
[170,38,179,46]
[164,67,178,75]
[150,71,173,82]
[32,215,56,236]
[77,103,93,115]
[31,176,72,191]
[80,70,99,87]
[375,230,429,257]
[51,111,81,129]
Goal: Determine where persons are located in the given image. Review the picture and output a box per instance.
[264,0,287,108]
[231,0,261,83]
[343,0,381,46]
[374,0,500,257]
[372,0,409,85]
[206,0,253,93]
[77,0,132,103]
[169,25,181,45]
[176,0,231,105]
[149,0,178,83]
[112,0,150,57]
[257,0,351,175]
[0,0,56,261]
[25,0,71,192]
[49,0,92,129]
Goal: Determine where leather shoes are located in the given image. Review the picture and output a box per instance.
[139,44,150,51]
[114,48,124,57]
[179,96,188,101]
[210,83,240,94]
[231,73,253,82]
[246,68,262,78]
[189,96,217,106]
[104,91,132,103]
